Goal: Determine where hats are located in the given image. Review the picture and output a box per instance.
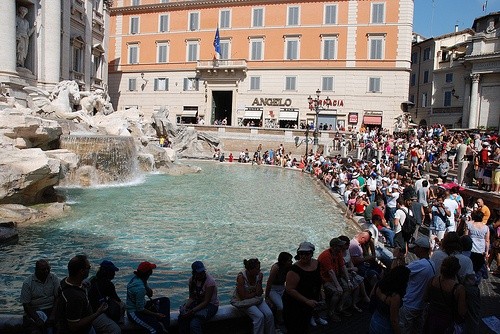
[192,261,207,273]
[297,242,315,253]
[100,260,119,272]
[408,236,431,249]
[349,245,361,258]
[138,262,156,273]
[330,237,347,247]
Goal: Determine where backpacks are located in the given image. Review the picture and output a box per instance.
[399,208,417,237]
[428,280,461,329]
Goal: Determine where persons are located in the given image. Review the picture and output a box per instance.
[21,255,170,334]
[236,258,276,334]
[266,230,483,334]
[160,136,168,147]
[16,7,37,67]
[247,120,278,128]
[215,149,233,162]
[176,261,219,334]
[299,115,500,275]
[197,117,204,124]
[214,117,227,126]
[253,144,297,167]
[285,122,297,129]
[240,149,249,163]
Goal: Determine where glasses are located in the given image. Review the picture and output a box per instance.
[40,266,52,271]
[299,251,313,256]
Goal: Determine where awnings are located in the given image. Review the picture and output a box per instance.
[181,110,198,117]
[279,111,298,121]
[244,111,263,119]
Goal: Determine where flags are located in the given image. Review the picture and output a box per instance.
[213,29,222,56]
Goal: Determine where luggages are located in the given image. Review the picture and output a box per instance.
[145,297,170,327]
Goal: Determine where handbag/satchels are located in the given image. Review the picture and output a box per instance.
[189,298,219,320]
[228,270,263,309]
[104,295,125,322]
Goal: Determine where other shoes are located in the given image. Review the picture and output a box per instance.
[316,317,328,325]
[310,315,317,326]
[277,321,287,333]
[337,308,352,316]
[329,314,341,322]
[362,295,371,304]
[351,304,363,313]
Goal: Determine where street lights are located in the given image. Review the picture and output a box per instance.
[294,125,312,163]
[306,89,331,145]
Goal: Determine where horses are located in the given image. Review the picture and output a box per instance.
[23,80,81,120]
